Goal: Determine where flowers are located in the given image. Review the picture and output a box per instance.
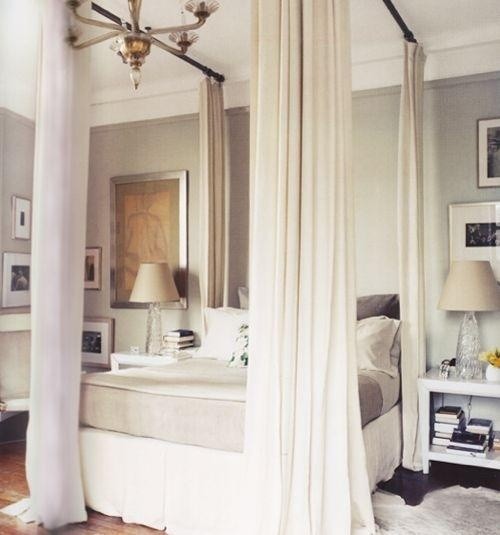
[438,260,500,379]
[480,348,499,366]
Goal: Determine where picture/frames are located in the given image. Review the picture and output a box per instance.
[2,253,31,309]
[109,169,190,312]
[11,196,29,241]
[476,117,500,190]
[81,317,116,370]
[447,202,500,284]
[84,247,101,291]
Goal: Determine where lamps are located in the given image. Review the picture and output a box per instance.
[64,0,219,90]
[128,262,181,355]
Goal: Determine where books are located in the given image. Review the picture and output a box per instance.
[164,330,195,351]
[448,431,490,458]
[432,407,466,447]
[467,417,493,436]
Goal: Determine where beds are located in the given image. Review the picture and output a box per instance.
[77,291,404,535]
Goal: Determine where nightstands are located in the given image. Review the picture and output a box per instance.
[111,349,189,372]
[418,367,500,476]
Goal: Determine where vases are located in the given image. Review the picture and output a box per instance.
[486,364,500,381]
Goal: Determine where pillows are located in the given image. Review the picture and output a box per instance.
[355,316,399,375]
[196,306,248,364]
